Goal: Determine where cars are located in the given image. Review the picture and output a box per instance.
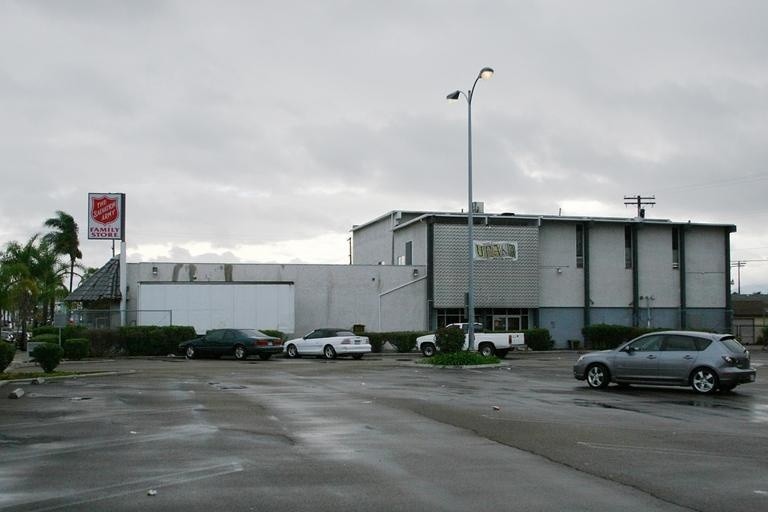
[1,329,31,343]
[573,330,757,395]
[283,328,372,360]
[178,329,284,361]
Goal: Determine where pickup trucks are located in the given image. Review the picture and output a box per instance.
[415,322,524,358]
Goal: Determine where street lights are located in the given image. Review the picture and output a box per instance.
[446,67,494,353]
